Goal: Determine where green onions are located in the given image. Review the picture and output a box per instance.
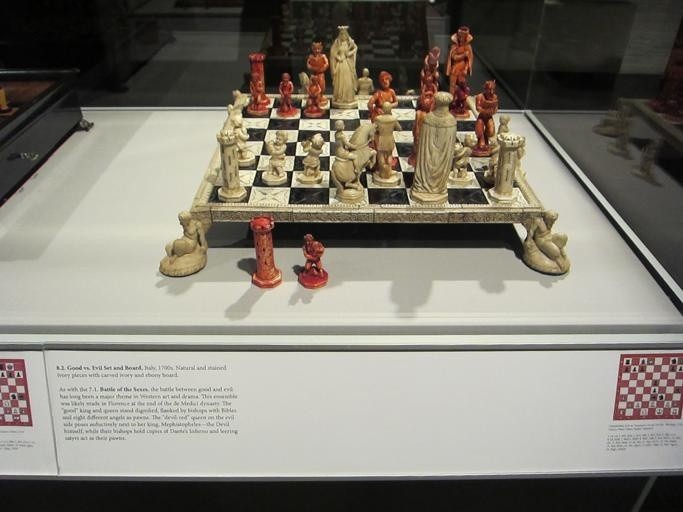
[190,93,546,234]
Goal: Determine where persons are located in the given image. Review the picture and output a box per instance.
[215,25,527,207]
